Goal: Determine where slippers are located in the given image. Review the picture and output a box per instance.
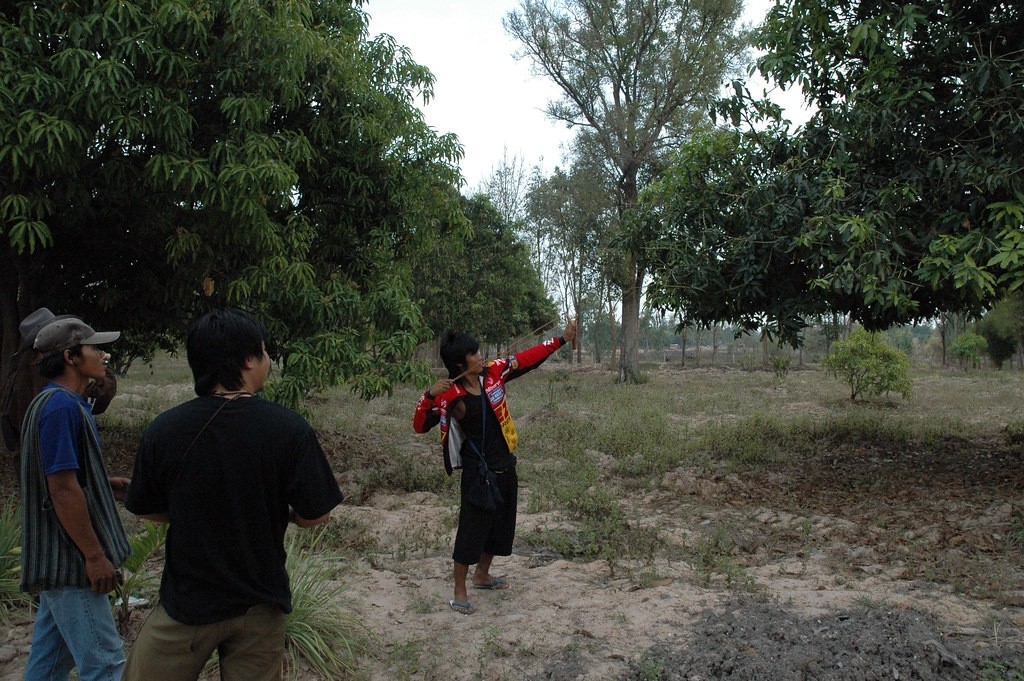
[449,599,476,615]
[473,576,512,592]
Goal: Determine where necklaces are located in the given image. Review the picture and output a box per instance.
[213,390,254,397]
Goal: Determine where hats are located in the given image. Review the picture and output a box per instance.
[10,307,78,359]
[32,317,122,360]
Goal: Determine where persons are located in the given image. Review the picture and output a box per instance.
[20,318,133,681]
[120,307,343,680]
[414,325,577,615]
[0,308,116,487]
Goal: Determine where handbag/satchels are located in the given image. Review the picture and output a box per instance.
[85,368,117,416]
[461,463,505,512]
